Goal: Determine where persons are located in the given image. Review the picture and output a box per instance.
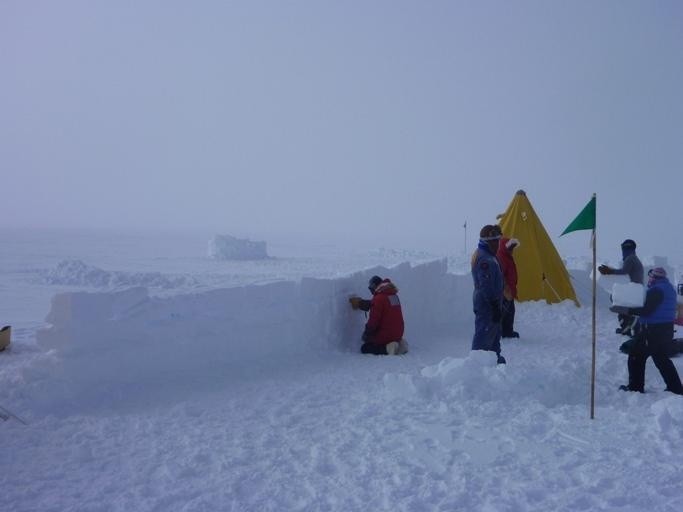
[471,224,505,363]
[599,239,683,395]
[495,239,520,338]
[350,277,408,354]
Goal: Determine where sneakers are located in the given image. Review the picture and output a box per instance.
[618,382,645,394]
[386,340,410,355]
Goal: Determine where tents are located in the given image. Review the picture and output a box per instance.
[472,190,582,307]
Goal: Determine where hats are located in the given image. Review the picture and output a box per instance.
[647,266,666,289]
[367,275,382,291]
[620,239,636,249]
[479,225,501,242]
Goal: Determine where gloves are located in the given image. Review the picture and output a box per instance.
[609,304,630,315]
[351,297,370,312]
[598,264,616,276]
[488,294,503,323]
